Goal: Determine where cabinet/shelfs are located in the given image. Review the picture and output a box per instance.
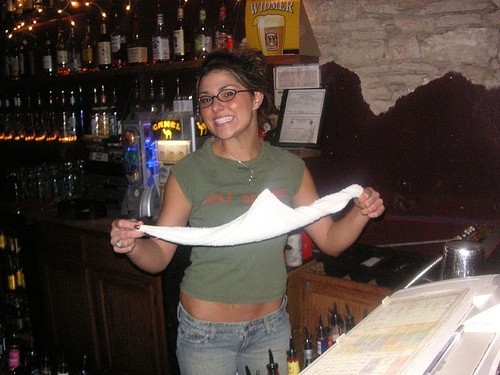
[301,278,376,333]
[2,221,163,375]
[1,0,322,160]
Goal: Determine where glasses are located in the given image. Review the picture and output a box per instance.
[195,89,250,109]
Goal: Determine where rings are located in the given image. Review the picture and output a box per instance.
[116,239,121,247]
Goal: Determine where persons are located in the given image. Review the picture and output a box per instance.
[109,52,385,375]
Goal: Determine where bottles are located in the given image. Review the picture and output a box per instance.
[193,0,213,58]
[152,5,170,62]
[0,10,147,147]
[0,294,92,375]
[131,78,168,114]
[214,0,234,51]
[234,307,360,375]
[172,5,190,62]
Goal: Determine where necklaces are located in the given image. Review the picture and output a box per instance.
[220,142,265,186]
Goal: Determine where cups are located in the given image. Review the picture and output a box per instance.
[0,159,88,211]
[440,241,486,280]
[255,14,286,57]
